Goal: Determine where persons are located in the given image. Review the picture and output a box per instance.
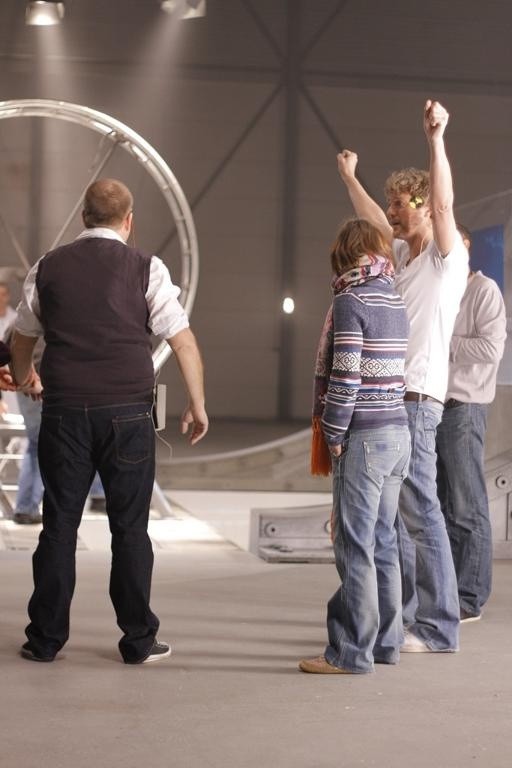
[436,224,508,622]
[337,99,470,652]
[9,177,209,664]
[1,267,106,523]
[299,221,411,674]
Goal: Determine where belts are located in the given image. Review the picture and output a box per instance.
[403,391,428,402]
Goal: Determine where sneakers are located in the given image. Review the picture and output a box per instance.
[90,499,105,512]
[142,641,171,662]
[13,512,43,524]
[400,629,455,653]
[20,641,53,662]
[300,655,361,673]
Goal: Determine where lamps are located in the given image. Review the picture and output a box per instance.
[159,0,206,20]
[24,0,66,27]
